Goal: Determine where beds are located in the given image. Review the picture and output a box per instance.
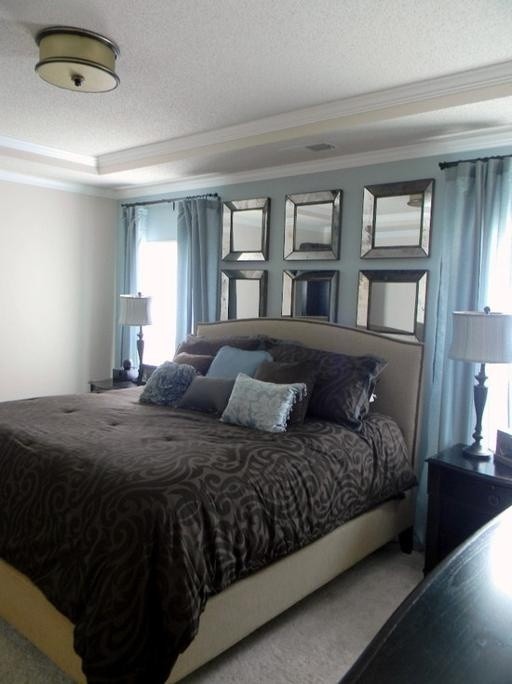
[0,318,425,684]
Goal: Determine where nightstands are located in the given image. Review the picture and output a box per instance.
[88,379,141,389]
[425,443,512,575]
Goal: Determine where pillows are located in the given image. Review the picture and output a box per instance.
[139,334,389,435]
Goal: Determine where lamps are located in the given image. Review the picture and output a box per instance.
[34,26,121,93]
[118,291,154,385]
[448,306,511,457]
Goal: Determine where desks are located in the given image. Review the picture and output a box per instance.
[339,507,512,683]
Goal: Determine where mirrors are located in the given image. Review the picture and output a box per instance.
[361,178,434,259]
[284,190,341,261]
[282,270,339,322]
[220,269,268,321]
[357,269,429,343]
[222,197,270,262]
